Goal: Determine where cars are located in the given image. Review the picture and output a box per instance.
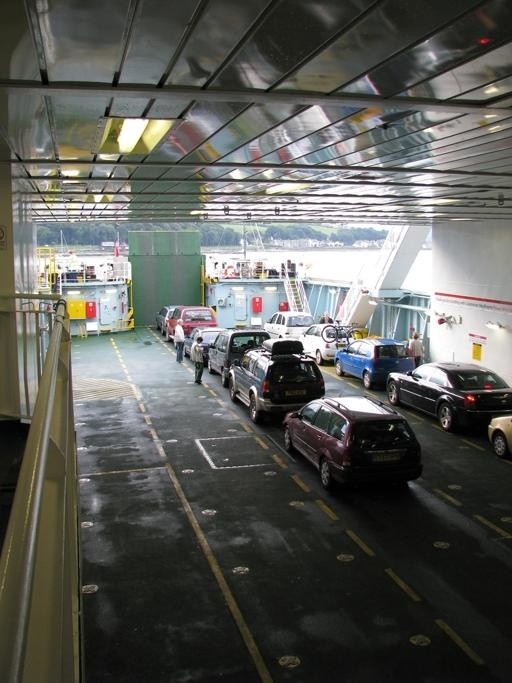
[282,393,421,487]
[334,337,416,388]
[487,414,512,457]
[156,303,356,387]
[386,361,511,434]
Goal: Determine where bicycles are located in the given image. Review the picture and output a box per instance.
[321,320,364,343]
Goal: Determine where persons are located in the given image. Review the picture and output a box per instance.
[409,333,425,367]
[191,337,204,384]
[320,312,333,323]
[175,319,185,363]
